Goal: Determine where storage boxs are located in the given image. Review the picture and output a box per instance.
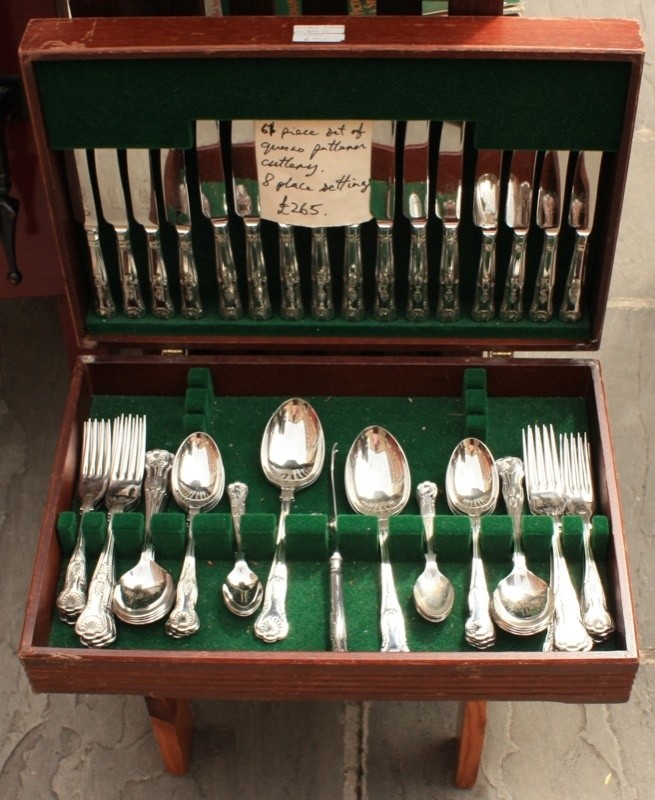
[16,15,647,705]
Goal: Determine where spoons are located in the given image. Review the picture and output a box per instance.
[111,449,176,624]
[488,454,555,636]
[413,478,454,623]
[344,424,411,652]
[444,436,499,650]
[165,398,326,652]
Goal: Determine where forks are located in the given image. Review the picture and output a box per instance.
[522,422,615,650]
[56,413,147,648]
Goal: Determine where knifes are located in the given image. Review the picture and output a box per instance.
[528,149,604,323]
[370,120,397,321]
[195,120,244,321]
[434,120,466,322]
[125,147,175,320]
[93,148,145,320]
[497,149,538,322]
[231,120,274,321]
[159,148,204,321]
[401,119,431,321]
[64,147,116,320]
[470,148,503,322]
